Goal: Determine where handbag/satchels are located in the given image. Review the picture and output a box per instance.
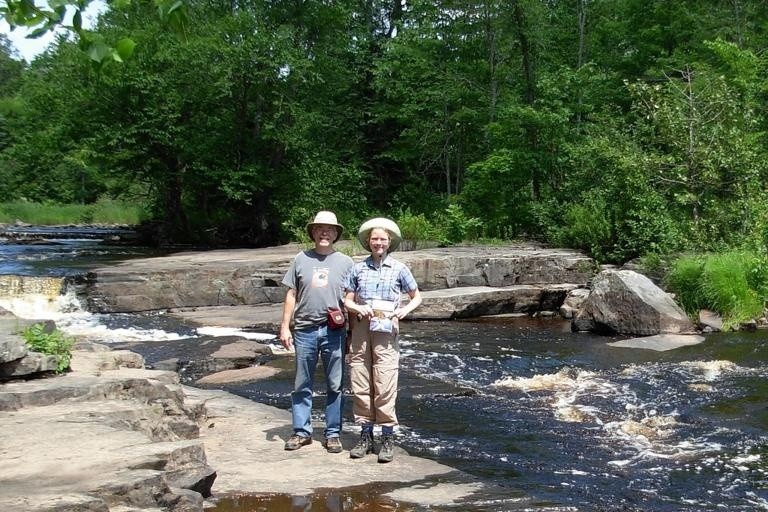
[327,309,345,331]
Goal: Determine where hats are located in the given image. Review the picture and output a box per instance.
[358,217,401,253]
[306,210,344,243]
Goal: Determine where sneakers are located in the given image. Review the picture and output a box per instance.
[325,435,343,453]
[378,433,394,463]
[350,432,374,458]
[284,435,312,450]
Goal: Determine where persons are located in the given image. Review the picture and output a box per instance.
[278,209,356,453]
[342,215,423,463]
